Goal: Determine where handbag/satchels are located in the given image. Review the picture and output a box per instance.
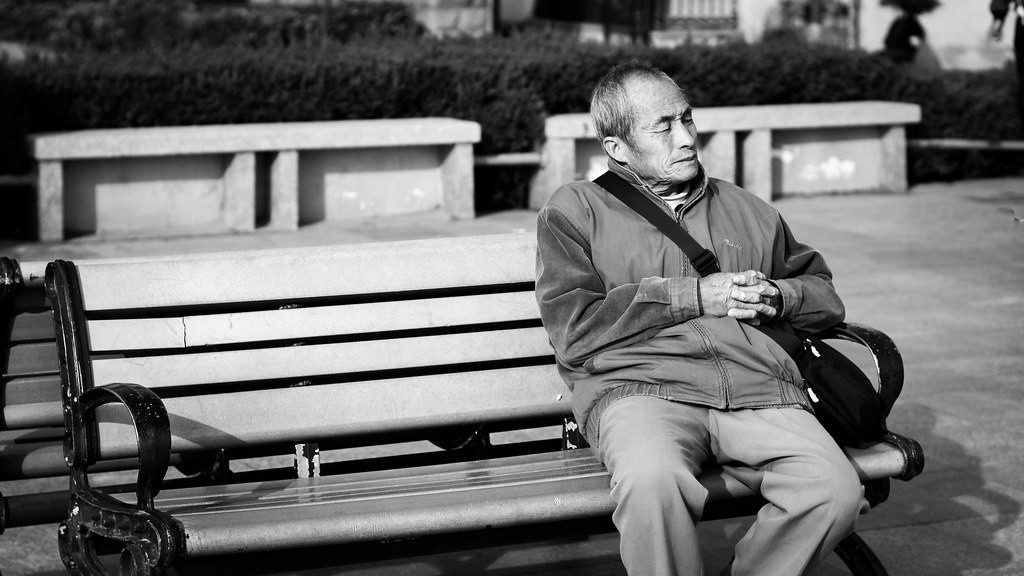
[800,338,888,450]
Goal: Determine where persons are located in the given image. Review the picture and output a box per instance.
[534,64,863,576]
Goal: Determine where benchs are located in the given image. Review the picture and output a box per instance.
[0,230,925,576]
